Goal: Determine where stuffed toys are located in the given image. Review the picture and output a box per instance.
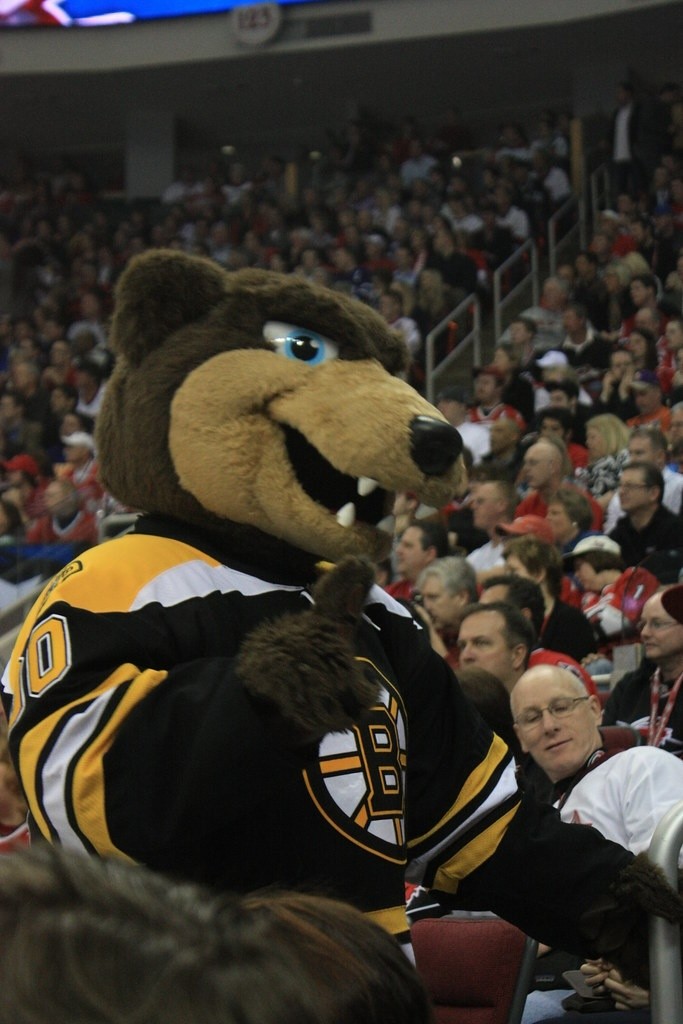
[0,250,683,1023]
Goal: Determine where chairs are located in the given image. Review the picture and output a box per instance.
[410,917,537,1024]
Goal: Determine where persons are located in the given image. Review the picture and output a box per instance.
[0,83,683,915]
[443,663,683,1023]
[1,848,438,1024]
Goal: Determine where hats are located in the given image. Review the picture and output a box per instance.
[536,350,568,370]
[562,535,621,560]
[473,364,507,384]
[494,513,556,546]
[61,429,94,451]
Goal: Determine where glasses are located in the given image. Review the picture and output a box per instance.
[636,619,678,632]
[515,695,588,732]
[611,479,646,492]
